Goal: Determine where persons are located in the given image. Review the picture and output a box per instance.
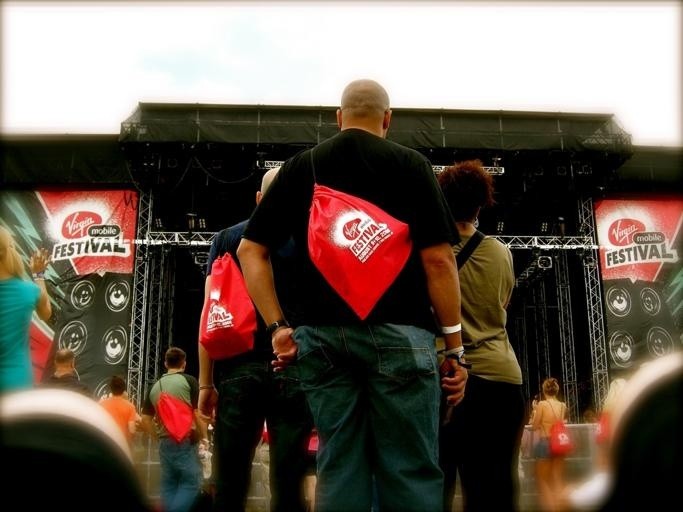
[0,226,51,400]
[526,352,683,512]
[427,159,525,512]
[236,79,468,512]
[0,348,212,512]
[197,165,314,512]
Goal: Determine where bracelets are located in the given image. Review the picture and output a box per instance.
[266,319,289,340]
[439,323,461,334]
[32,271,46,280]
[443,346,465,360]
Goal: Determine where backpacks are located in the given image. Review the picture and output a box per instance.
[307,184,413,320]
[549,420,574,455]
[200,253,257,358]
[157,392,194,443]
